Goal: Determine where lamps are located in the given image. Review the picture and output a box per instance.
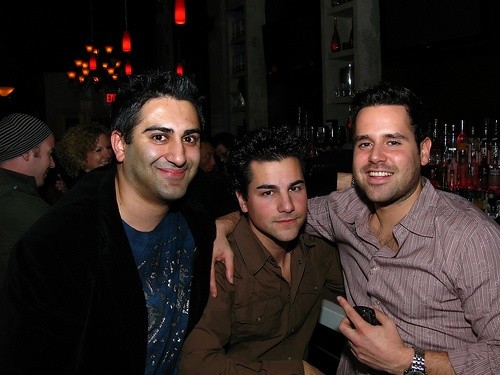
[0,0,187,96]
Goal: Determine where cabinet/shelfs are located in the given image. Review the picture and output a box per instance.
[208,0,268,135]
[320,0,381,125]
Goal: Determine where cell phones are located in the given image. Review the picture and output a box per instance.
[350,306,378,328]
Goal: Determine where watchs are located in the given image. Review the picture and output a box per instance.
[403,346,427,375]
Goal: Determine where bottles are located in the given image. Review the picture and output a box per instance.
[232,42,244,73]
[232,17,244,41]
[238,119,246,136]
[293,105,354,158]
[418,117,500,220]
[349,22,353,49]
[344,63,354,96]
[331,17,341,52]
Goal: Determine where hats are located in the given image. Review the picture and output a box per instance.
[0,113,52,162]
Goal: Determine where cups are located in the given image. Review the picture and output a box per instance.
[335,82,345,98]
[342,42,348,50]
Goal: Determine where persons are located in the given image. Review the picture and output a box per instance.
[0,70,241,375]
[176,128,346,375]
[211,85,500,375]
[199,131,240,179]
[0,113,113,288]
